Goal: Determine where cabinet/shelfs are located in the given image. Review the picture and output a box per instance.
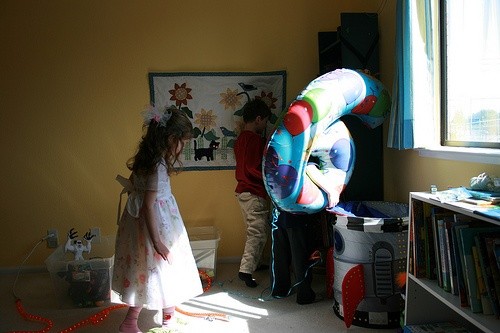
[402,190,500,333]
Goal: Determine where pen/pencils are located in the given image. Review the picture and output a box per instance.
[461,199,477,205]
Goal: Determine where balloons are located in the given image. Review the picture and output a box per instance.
[261,69,393,214]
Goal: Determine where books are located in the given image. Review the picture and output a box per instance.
[431,187,500,222]
[409,199,500,320]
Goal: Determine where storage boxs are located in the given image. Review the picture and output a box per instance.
[187,225,222,277]
[45,243,116,307]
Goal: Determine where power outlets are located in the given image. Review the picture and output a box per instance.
[90,228,100,243]
[48,229,57,248]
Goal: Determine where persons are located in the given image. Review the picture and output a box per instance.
[110,105,204,333]
[233,97,271,288]
[271,202,326,306]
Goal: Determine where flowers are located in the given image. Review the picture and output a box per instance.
[141,104,168,125]
